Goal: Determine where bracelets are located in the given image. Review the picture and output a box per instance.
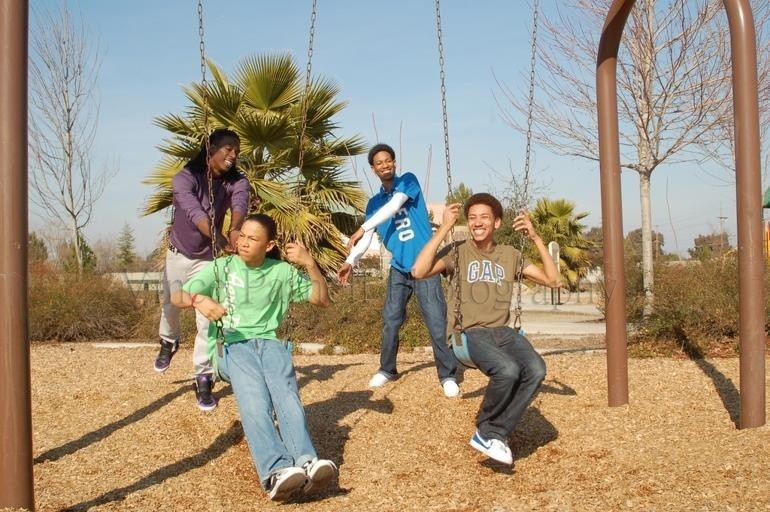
[191,292,200,307]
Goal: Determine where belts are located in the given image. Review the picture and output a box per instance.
[169,242,182,253]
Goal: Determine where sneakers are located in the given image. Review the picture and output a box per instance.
[154,335,179,372]
[195,372,217,411]
[443,380,460,398]
[368,372,389,387]
[468,427,514,467]
[299,459,335,497]
[268,465,307,501]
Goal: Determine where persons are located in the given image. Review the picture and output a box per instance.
[409,192,566,466]
[154,128,250,411]
[170,212,336,502]
[336,143,461,400]
